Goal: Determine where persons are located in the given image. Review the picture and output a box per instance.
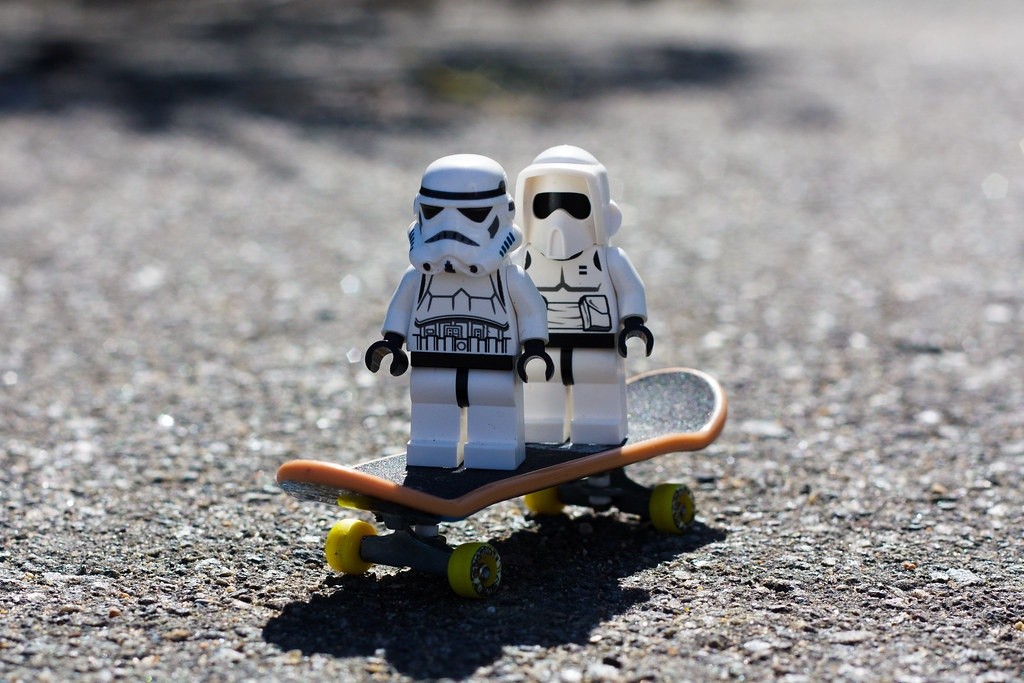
[513,144,653,447]
[363,152,556,473]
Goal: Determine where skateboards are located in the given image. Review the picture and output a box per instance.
[274,366,729,597]
